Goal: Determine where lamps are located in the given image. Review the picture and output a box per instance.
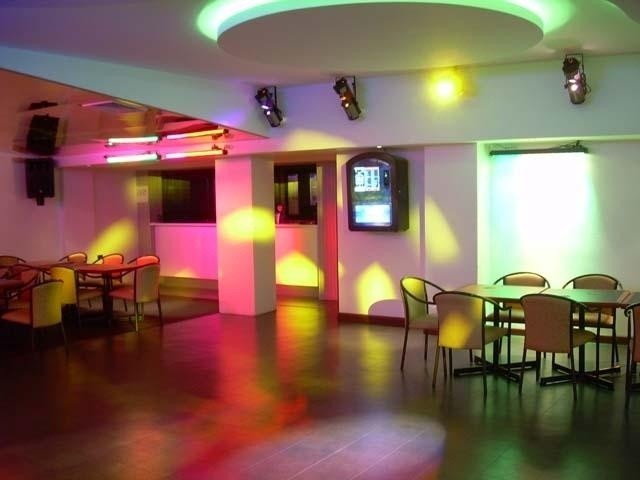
[328,68,362,122]
[560,49,590,107]
[254,81,285,132]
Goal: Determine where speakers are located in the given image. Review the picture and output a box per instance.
[26,158,55,206]
[26,115,59,154]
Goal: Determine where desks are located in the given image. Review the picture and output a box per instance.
[539,286,630,396]
[624,288,640,308]
[455,283,547,393]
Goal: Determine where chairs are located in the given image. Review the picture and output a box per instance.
[621,301,640,414]
[0,249,167,351]
[398,275,476,382]
[432,290,516,400]
[518,294,597,407]
[561,272,623,365]
[480,272,553,362]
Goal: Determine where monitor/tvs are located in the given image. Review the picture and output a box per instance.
[346,152,393,205]
